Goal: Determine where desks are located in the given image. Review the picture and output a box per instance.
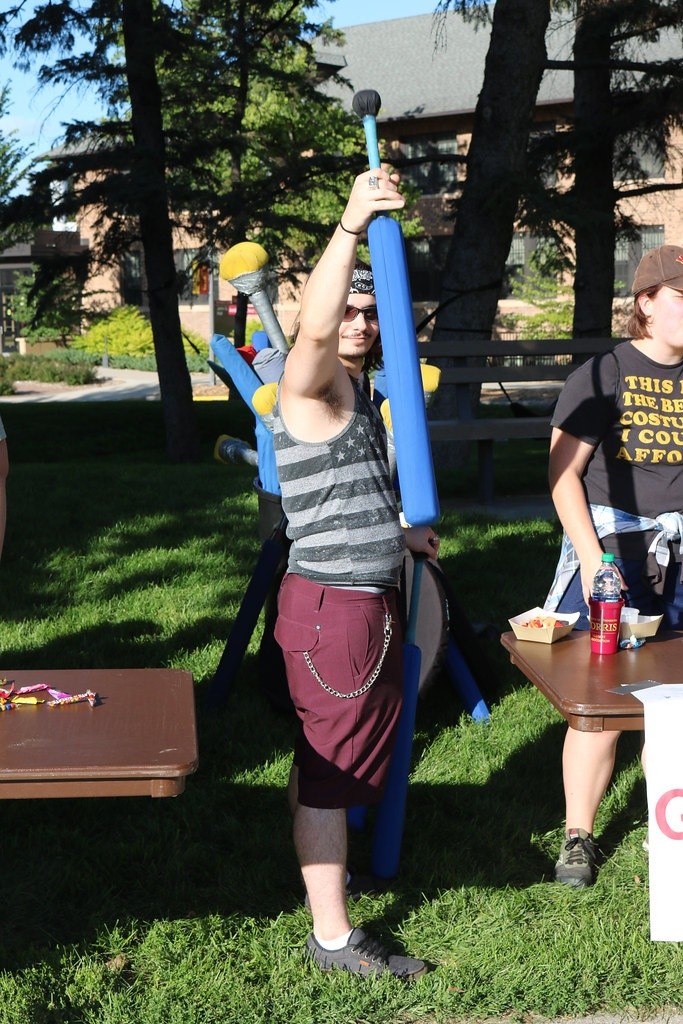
[500,627,683,732]
[0,668,197,802]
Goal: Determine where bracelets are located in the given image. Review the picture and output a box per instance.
[340,220,365,235]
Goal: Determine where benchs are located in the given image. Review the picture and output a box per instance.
[418,337,637,507]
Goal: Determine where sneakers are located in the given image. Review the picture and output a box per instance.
[305,874,381,915]
[305,927,428,980]
[553,828,599,887]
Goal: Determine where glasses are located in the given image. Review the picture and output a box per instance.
[342,305,379,326]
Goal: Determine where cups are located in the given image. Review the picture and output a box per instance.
[588,597,625,655]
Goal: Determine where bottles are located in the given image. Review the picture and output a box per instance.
[594,553,621,602]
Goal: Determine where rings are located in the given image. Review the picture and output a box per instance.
[367,175,381,191]
[430,535,439,542]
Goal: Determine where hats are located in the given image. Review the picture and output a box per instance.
[632,245,683,296]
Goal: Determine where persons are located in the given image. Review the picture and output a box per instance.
[546,245,682,890]
[271,164,440,980]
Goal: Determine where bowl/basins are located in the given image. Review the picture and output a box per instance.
[620,608,639,624]
